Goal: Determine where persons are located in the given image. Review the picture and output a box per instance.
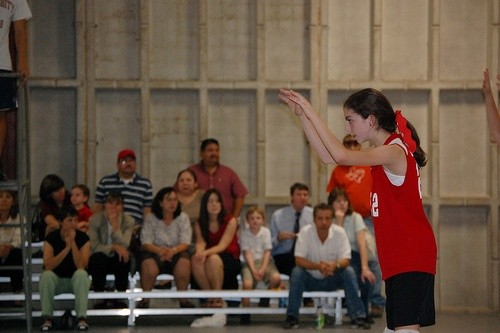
[278,85,437,332]
[37,205,92,333]
[0,188,31,308]
[269,182,317,274]
[170,168,208,226]
[86,189,137,312]
[135,187,196,310]
[327,188,378,323]
[328,133,385,315]
[478,67,500,148]
[68,183,93,233]
[189,188,241,314]
[283,203,373,330]
[34,173,78,240]
[184,138,248,217]
[92,148,153,252]
[238,207,281,325]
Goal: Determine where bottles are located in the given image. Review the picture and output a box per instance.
[317,306,324,330]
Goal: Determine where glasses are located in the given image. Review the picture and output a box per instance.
[343,140,358,147]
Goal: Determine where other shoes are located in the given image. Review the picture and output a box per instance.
[351,317,371,330]
[136,302,150,309]
[113,301,129,309]
[179,300,193,308]
[369,304,383,318]
[93,301,107,310]
[78,322,88,331]
[258,297,270,308]
[203,299,223,308]
[240,313,251,325]
[41,321,51,331]
[154,281,171,290]
[282,316,299,329]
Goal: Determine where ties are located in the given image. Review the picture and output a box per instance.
[290,211,301,252]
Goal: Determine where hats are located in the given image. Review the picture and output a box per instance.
[118,149,136,160]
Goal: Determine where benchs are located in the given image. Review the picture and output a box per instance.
[0,234,362,327]
